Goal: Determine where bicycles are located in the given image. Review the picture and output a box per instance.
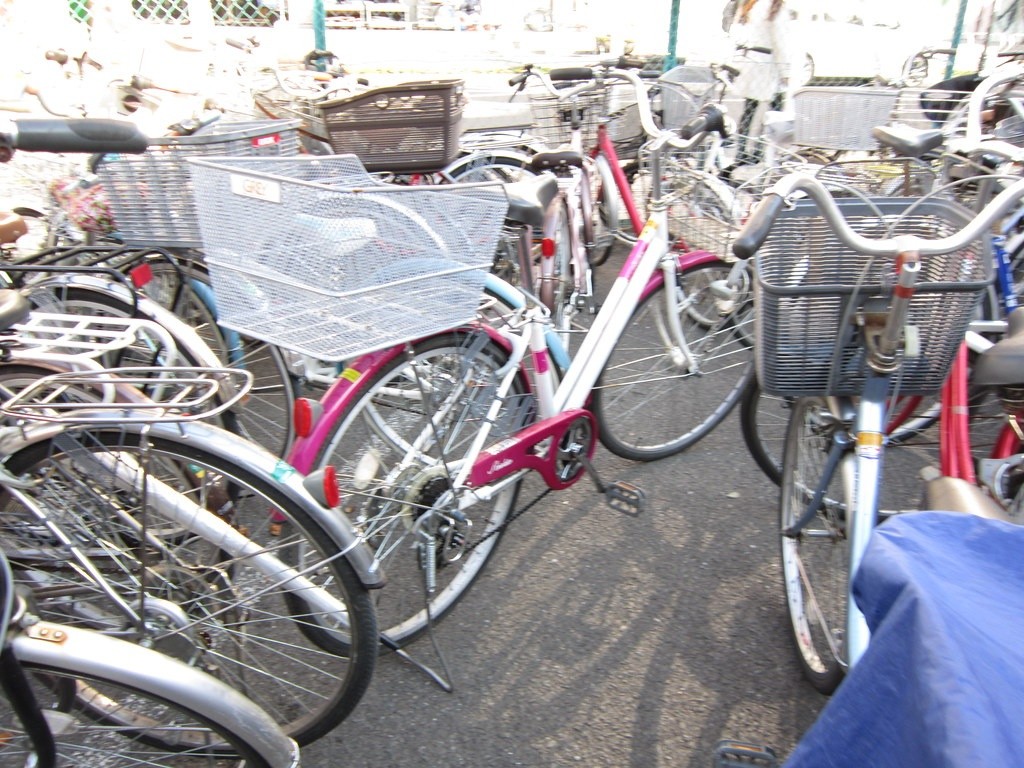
[708,156,1023,768]
[0,37,1024,768]
[175,64,783,697]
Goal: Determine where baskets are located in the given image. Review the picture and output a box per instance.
[638,128,810,263]
[315,79,465,174]
[993,115,1024,149]
[737,164,882,218]
[658,65,720,133]
[757,196,996,395]
[531,85,612,144]
[184,153,511,362]
[90,117,304,249]
[731,54,790,100]
[892,88,975,139]
[793,85,898,152]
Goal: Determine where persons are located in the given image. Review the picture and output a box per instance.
[730,0,789,162]
